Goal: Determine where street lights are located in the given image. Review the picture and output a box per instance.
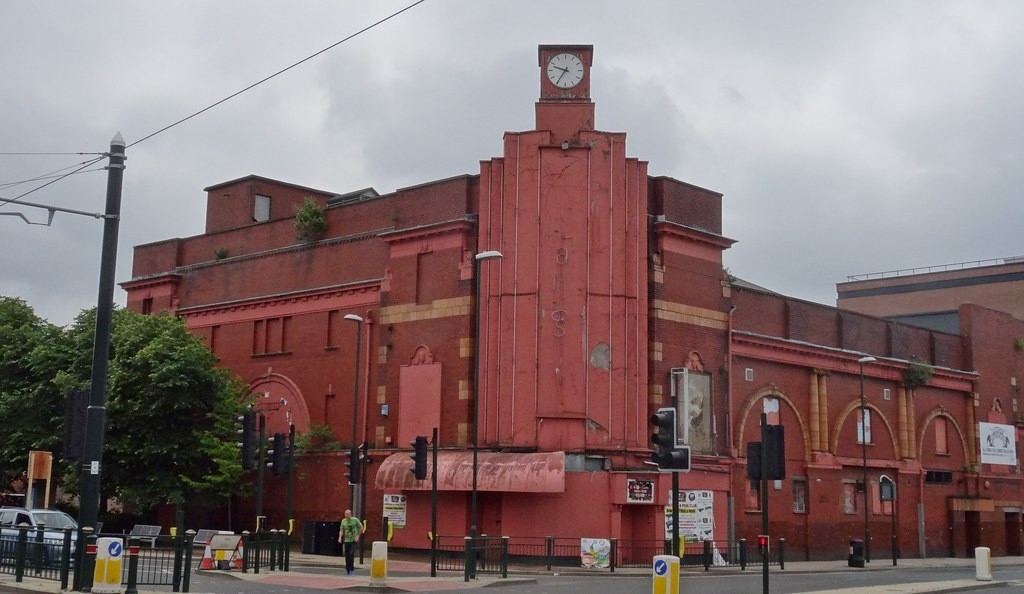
[344,314,363,517]
[470,250,504,578]
[858,356,876,563]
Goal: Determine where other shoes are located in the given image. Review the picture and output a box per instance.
[347,570,353,577]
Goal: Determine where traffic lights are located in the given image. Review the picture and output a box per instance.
[343,446,359,484]
[267,433,286,474]
[237,406,255,469]
[650,408,690,473]
[409,436,427,480]
[759,535,769,556]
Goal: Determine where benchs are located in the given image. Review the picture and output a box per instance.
[126,524,163,548]
[185,529,234,547]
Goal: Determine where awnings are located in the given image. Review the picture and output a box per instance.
[375,450,567,493]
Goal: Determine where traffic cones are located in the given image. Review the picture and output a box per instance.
[200,538,216,570]
[235,541,243,569]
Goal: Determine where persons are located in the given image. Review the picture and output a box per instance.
[338,509,363,575]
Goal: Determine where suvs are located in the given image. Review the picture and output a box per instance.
[0,506,78,565]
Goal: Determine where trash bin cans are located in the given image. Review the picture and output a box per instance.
[848,539,864,568]
[301,520,342,556]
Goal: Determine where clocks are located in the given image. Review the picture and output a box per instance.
[546,51,586,90]
